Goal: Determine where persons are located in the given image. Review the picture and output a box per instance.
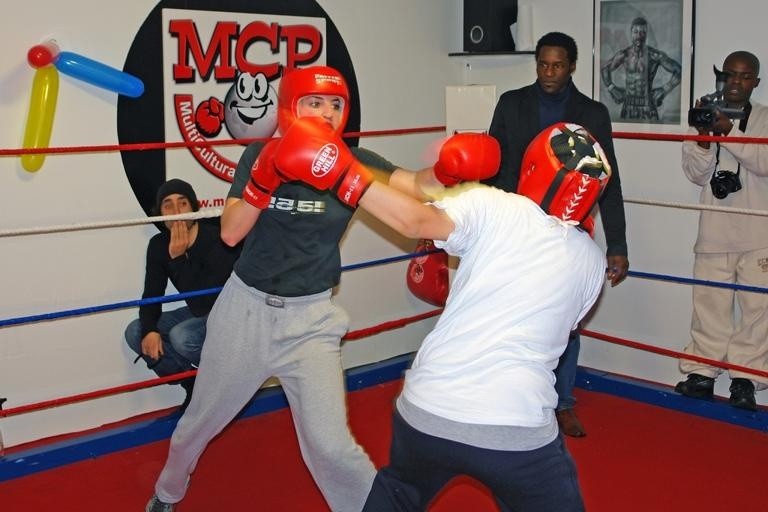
[274,116,607,512]
[599,15,681,119]
[142,62,503,512]
[486,30,630,437]
[122,177,242,411]
[674,50,767,409]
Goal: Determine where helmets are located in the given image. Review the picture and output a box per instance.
[516,122,613,224]
[278,66,350,137]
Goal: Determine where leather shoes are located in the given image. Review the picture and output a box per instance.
[555,408,587,437]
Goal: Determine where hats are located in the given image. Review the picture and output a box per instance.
[156,179,199,215]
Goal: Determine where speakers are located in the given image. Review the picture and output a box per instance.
[464,0,518,52]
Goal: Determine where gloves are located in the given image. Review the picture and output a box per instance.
[433,133,502,187]
[241,115,377,210]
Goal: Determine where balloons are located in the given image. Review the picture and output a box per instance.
[55,49,144,98]
[16,63,59,173]
[27,44,55,68]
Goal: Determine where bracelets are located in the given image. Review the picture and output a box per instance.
[606,83,614,92]
[661,82,672,93]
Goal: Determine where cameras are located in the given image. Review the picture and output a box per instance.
[710,169,742,199]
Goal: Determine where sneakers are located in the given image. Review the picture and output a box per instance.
[729,378,757,412]
[675,373,715,400]
